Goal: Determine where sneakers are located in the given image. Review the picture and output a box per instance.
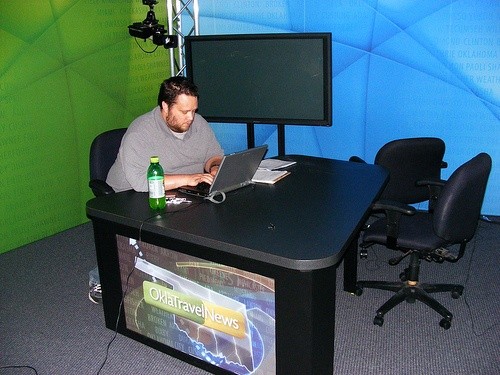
[89,280,102,305]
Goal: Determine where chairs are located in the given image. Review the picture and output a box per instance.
[347,137,447,263]
[89,128,128,196]
[355,152,493,330]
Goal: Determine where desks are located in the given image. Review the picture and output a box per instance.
[86,154,392,375]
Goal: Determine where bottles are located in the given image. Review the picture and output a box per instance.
[147,156,166,209]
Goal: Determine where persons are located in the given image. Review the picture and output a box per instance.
[88,76,224,305]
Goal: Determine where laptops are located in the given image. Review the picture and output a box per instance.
[177,144,268,197]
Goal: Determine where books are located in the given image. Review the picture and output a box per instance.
[252,158,297,184]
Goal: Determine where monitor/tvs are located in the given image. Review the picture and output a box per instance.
[185,33,332,127]
